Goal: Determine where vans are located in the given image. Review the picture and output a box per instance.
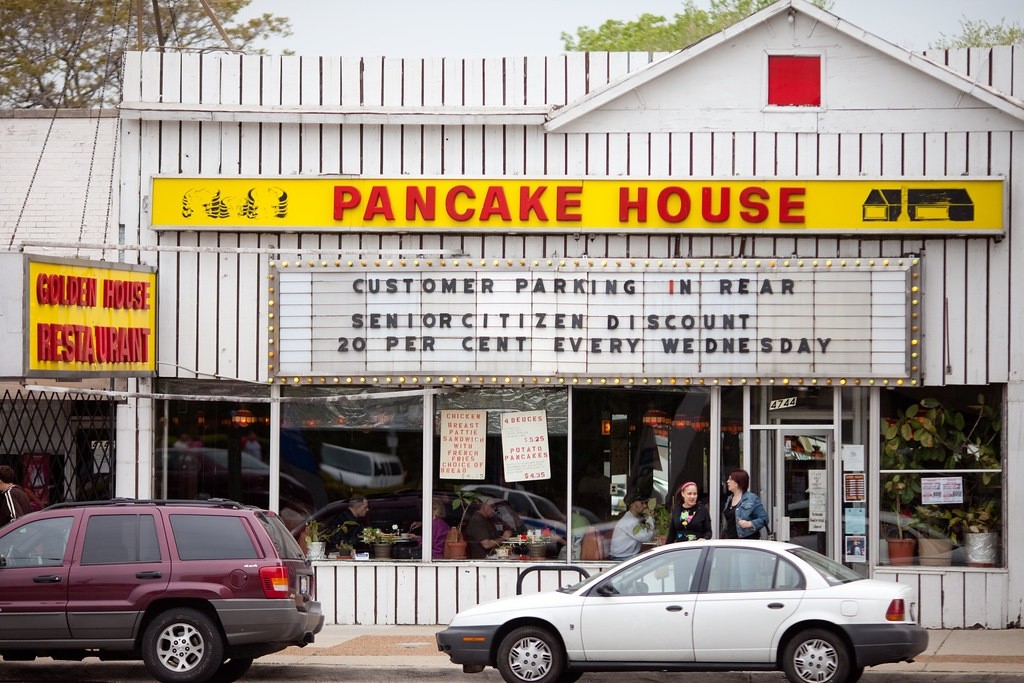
[318,442,407,495]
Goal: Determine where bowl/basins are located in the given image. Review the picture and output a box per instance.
[401,533,409,538]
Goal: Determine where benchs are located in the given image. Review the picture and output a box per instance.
[580,527,613,559]
[444,529,471,560]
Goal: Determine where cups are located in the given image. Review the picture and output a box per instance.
[363,528,370,541]
[535,529,541,539]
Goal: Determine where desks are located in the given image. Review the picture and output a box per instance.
[502,541,553,556]
[358,539,416,543]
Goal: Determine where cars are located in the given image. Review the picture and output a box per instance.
[435,539,930,683]
[461,484,573,549]
[88,448,312,512]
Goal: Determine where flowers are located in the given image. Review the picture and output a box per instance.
[522,527,557,544]
[357,527,400,544]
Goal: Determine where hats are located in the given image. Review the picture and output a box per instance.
[624,491,649,505]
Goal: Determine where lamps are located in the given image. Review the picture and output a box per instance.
[645,409,743,438]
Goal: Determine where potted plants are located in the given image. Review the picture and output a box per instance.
[447,483,485,558]
[336,540,353,556]
[880,391,1001,567]
[633,496,676,545]
[301,505,359,555]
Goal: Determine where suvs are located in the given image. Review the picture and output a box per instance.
[292,488,526,562]
[0,498,325,683]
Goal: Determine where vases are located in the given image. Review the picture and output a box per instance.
[373,544,394,557]
[527,544,548,557]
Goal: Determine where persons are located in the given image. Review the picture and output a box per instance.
[607,491,655,561]
[22,488,44,512]
[0,466,31,528]
[410,498,450,559]
[720,469,769,590]
[279,488,314,520]
[664,482,712,593]
[463,495,512,559]
[88,480,109,501]
[327,493,369,553]
[558,495,599,560]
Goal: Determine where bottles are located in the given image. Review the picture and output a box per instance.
[527,530,534,536]
